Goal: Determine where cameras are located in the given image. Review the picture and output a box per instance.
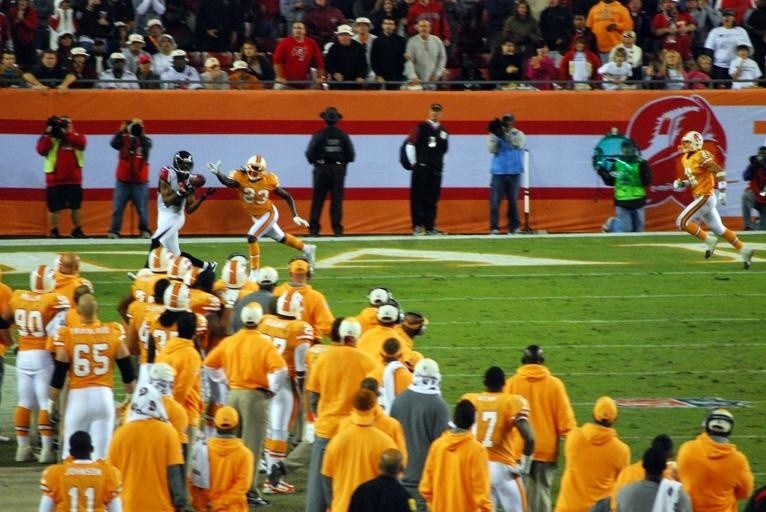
[123,120,143,137]
[749,154,766,168]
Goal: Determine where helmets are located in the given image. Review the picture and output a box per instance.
[172,151,192,174]
[245,155,265,181]
[276,291,303,318]
[147,248,192,311]
[414,358,442,380]
[681,131,703,153]
[29,265,55,293]
[220,260,248,289]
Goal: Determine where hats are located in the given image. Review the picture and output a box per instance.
[149,362,177,382]
[257,266,278,286]
[322,17,374,54]
[361,377,381,396]
[214,407,238,430]
[320,108,341,121]
[705,409,733,436]
[350,403,375,425]
[733,41,753,56]
[338,317,360,338]
[368,289,428,330]
[289,259,308,274]
[239,302,263,327]
[56,19,247,70]
[592,397,616,423]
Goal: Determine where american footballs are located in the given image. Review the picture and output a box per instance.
[189,174,205,187]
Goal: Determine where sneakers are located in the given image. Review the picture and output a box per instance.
[207,261,217,273]
[704,237,717,259]
[246,439,301,508]
[71,228,85,238]
[108,233,117,239]
[305,245,315,271]
[141,231,149,238]
[602,216,614,232]
[0,436,62,463]
[742,248,755,269]
[50,229,63,238]
[127,272,135,280]
[491,228,526,234]
[413,226,446,236]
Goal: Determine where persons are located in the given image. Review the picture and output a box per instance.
[1,0,765,92]
[105,113,152,239]
[403,102,450,237]
[304,104,355,235]
[486,113,527,236]
[205,152,317,273]
[671,130,756,271]
[593,140,653,233]
[36,112,89,238]
[417,338,766,512]
[741,145,766,231]
[141,147,217,273]
[0,245,452,512]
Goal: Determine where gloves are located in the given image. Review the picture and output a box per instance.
[718,192,726,206]
[176,182,192,197]
[292,216,309,227]
[200,187,215,200]
[206,161,221,174]
[506,464,529,478]
[673,178,682,189]
[46,398,61,424]
[115,394,131,416]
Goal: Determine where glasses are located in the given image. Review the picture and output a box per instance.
[623,34,635,37]
